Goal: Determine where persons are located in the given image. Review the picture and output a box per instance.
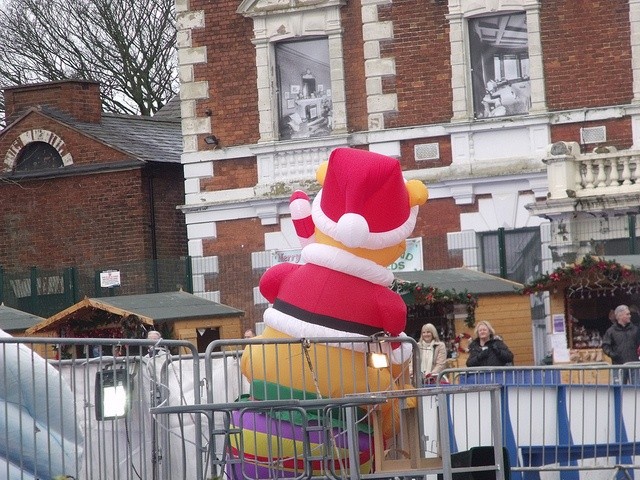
[143,331,171,360]
[417,324,447,385]
[599,304,640,365]
[464,320,515,368]
[243,329,255,341]
[224,149,430,480]
